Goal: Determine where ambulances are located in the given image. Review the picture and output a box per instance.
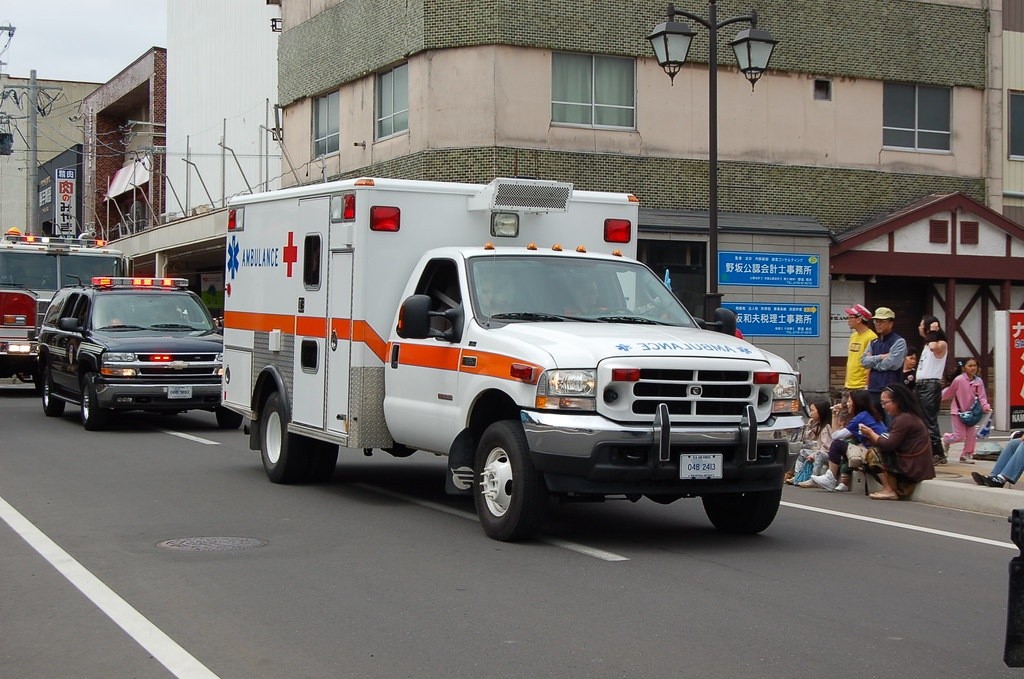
[221,177,806,541]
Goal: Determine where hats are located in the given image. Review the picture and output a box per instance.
[845,304,872,322]
[871,307,895,320]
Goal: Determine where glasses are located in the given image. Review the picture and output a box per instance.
[879,400,893,405]
[847,317,857,320]
[873,319,886,324]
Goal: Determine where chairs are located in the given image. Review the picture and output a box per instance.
[432,287,587,333]
[9,266,29,289]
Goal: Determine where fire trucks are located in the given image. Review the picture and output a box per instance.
[0,228,133,392]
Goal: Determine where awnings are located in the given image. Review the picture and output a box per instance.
[103,157,150,201]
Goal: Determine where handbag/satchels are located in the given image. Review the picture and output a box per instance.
[865,449,903,474]
[958,397,982,426]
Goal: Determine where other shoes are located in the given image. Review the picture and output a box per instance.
[869,492,899,499]
[972,472,1006,488]
[933,455,946,465]
[784,474,795,485]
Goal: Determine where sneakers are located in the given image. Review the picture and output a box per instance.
[960,456,975,464]
[942,433,949,453]
[798,477,820,488]
[811,469,837,491]
[835,483,849,491]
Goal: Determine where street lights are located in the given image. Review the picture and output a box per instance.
[645,2,780,329]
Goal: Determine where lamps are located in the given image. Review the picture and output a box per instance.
[838,274,845,281]
[869,275,877,283]
[69,113,87,122]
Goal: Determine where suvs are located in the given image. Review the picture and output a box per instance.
[37,276,243,431]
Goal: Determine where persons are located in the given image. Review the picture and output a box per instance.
[911,315,947,466]
[843,303,878,391]
[726,308,744,340]
[940,357,993,464]
[896,348,917,392]
[971,433,1024,490]
[861,307,908,434]
[784,381,936,500]
[564,278,605,318]
